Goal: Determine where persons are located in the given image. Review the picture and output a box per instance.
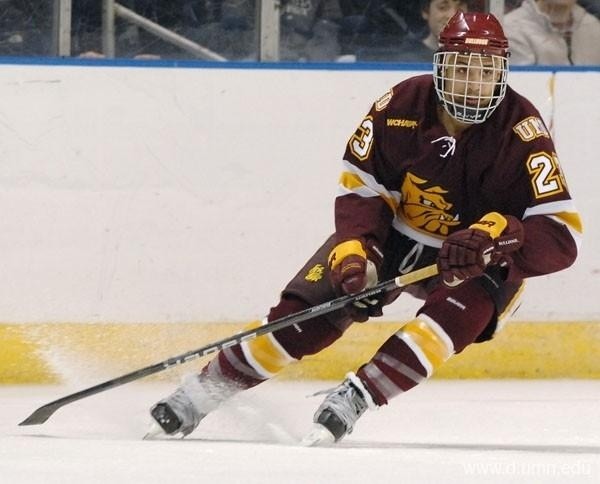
[148,11,588,443]
[0,0,600,68]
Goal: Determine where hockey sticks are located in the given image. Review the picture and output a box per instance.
[19,252,503,424]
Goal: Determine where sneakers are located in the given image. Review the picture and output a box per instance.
[150,374,216,434]
[315,374,374,442]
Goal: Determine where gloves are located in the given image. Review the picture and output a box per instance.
[328,238,386,323]
[436,211,525,291]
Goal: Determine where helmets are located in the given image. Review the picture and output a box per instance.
[432,10,510,125]
